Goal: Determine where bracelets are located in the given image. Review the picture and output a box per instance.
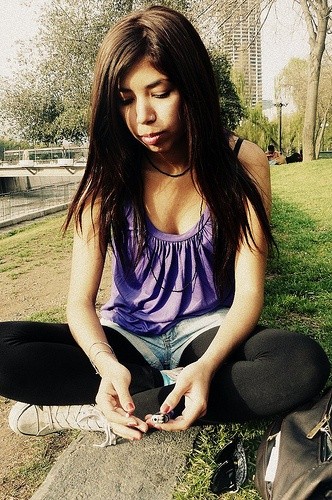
[89,341,114,374]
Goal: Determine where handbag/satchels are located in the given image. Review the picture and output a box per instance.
[255,387,332,500]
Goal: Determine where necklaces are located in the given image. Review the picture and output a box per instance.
[144,148,204,292]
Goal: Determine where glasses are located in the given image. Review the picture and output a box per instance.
[210,438,248,494]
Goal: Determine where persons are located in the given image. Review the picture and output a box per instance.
[271,149,303,165]
[0,8,331,438]
[265,145,279,161]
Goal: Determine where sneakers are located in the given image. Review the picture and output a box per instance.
[6,401,110,438]
[163,368,184,382]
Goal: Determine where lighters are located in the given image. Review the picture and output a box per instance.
[151,410,174,424]
